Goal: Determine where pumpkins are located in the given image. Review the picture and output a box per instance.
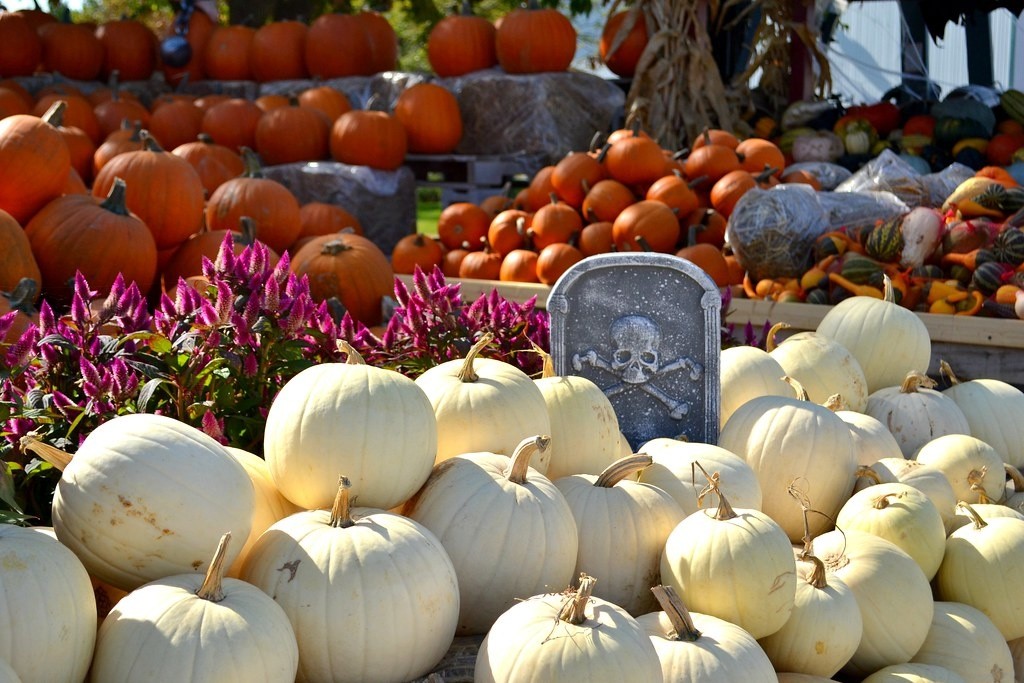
[0,0,1024,683]
